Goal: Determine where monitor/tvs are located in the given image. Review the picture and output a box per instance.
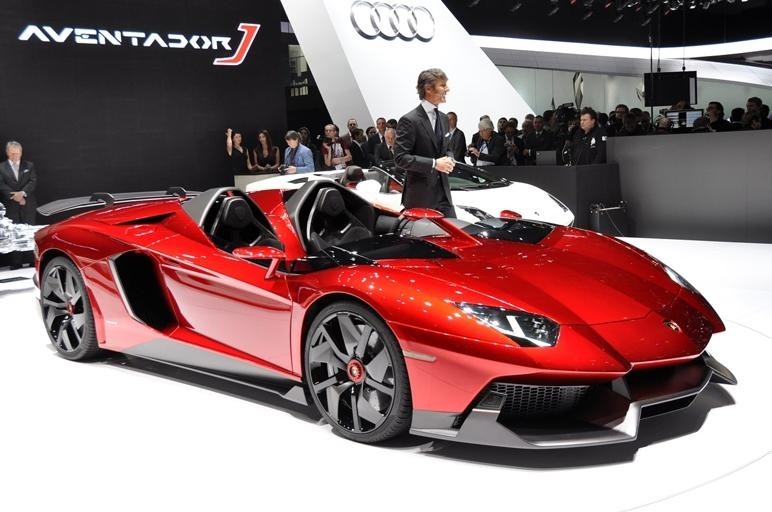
[664,109,704,129]
[644,71,697,107]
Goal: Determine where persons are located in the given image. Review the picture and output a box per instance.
[321,117,398,173]
[392,68,457,220]
[226,128,279,173]
[469,106,581,166]
[673,97,771,131]
[447,112,467,164]
[0,139,37,270]
[562,107,607,166]
[598,104,654,135]
[278,128,318,173]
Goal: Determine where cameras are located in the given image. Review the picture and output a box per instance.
[317,135,332,143]
[466,146,477,157]
[279,164,289,175]
[505,144,513,152]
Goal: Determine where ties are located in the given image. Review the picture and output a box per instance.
[434,108,442,153]
[388,146,393,159]
